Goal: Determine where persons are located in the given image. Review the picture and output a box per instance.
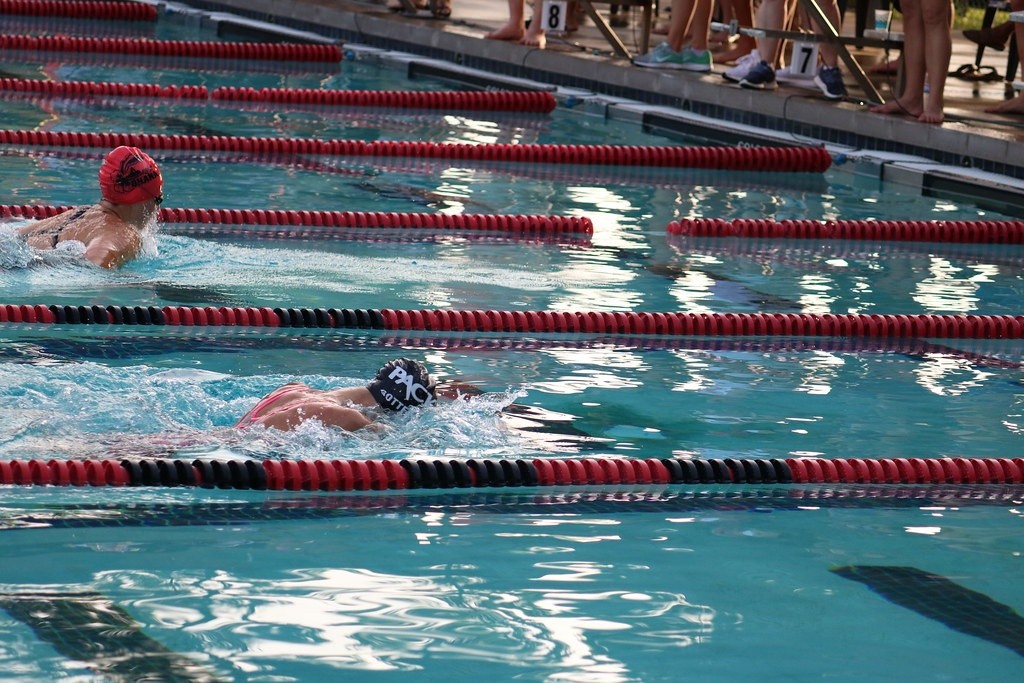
[484,0,546,45]
[387,0,452,17]
[721,0,868,97]
[963,0,1024,113]
[869,0,952,122]
[14,146,163,270]
[633,0,756,71]
[525,0,578,32]
[609,0,630,28]
[234,359,436,432]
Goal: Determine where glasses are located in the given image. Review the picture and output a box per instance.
[152,195,163,205]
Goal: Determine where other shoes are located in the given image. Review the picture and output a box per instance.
[430,0,452,20]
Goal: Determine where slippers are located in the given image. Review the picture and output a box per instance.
[947,63,1004,82]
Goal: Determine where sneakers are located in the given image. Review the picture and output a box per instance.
[814,64,845,99]
[739,61,779,90]
[630,42,714,72]
[721,49,760,83]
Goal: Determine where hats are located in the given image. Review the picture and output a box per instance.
[368,357,437,413]
[99,146,162,203]
